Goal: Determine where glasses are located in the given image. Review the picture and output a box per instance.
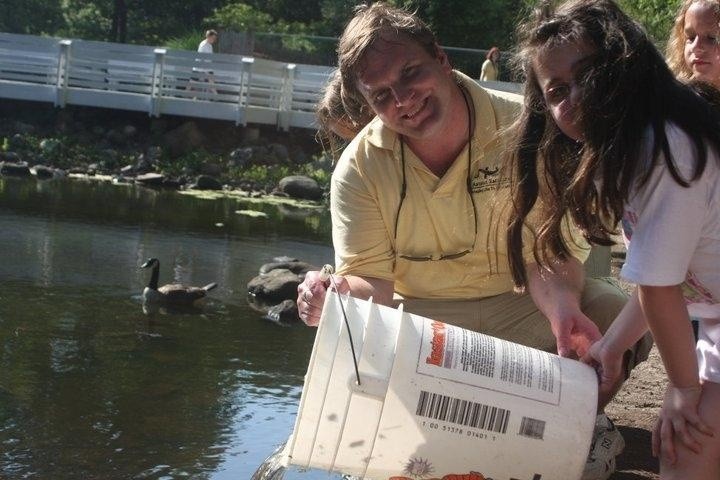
[544,64,595,111]
[393,178,477,262]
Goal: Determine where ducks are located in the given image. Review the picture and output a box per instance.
[140,257,218,302]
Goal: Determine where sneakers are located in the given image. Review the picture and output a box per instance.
[582,414,625,479]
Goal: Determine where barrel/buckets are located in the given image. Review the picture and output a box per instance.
[283,285,599,480]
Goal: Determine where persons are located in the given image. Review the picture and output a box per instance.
[660,1,719,92]
[296,1,648,410]
[313,70,378,166]
[186,27,220,94]
[485,2,720,480]
[480,44,502,83]
